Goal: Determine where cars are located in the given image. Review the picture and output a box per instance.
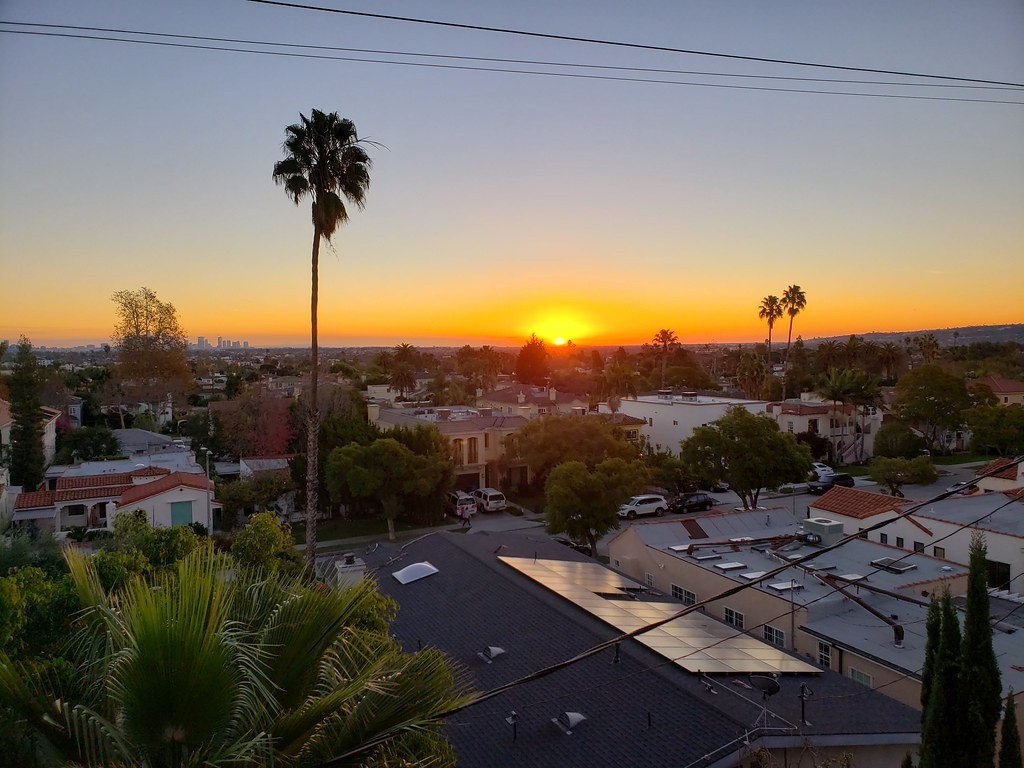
[945,481,979,496]
[551,536,591,557]
[700,478,730,492]
[807,462,834,479]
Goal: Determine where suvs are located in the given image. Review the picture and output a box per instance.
[806,472,856,495]
[616,494,669,519]
[469,488,507,513]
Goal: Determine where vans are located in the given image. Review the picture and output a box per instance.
[445,488,478,517]
[668,492,713,514]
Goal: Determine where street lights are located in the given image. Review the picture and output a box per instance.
[204,449,217,535]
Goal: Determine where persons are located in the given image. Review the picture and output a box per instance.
[459,507,465,522]
[462,507,472,526]
